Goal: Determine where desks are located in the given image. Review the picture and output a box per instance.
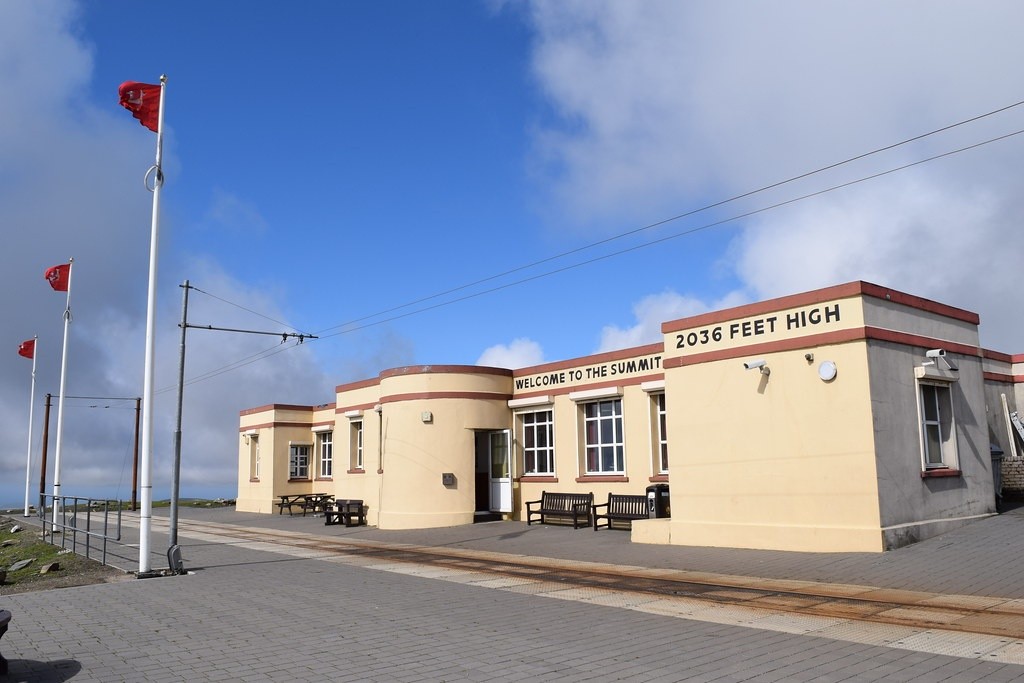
[277,494,325,515]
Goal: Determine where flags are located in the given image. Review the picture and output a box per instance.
[18,339,35,359]
[45,264,70,291]
[118,80,161,133]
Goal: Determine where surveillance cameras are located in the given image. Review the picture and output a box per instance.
[744,360,768,369]
[925,348,946,358]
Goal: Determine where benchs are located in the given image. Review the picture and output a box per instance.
[593,493,663,531]
[525,491,595,529]
[324,500,364,527]
[276,502,333,517]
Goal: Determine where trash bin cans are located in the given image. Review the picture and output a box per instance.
[990,443,1005,510]
[646,483,671,519]
